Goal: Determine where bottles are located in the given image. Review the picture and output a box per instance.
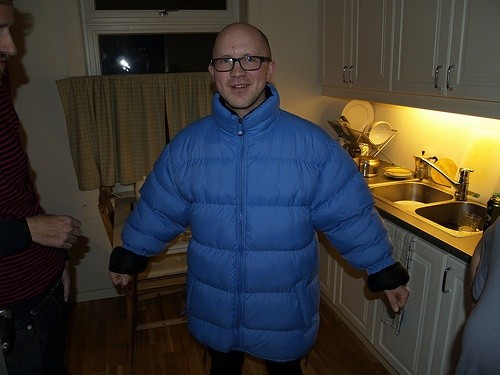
[483,196,500,235]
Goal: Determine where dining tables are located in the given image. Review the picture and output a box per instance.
[113,197,192,375]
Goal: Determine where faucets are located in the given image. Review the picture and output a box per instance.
[412,154,475,202]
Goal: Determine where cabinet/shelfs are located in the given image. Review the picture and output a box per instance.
[390,0,500,119]
[373,219,468,375]
[319,0,392,105]
[314,216,397,346]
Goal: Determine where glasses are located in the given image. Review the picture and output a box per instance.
[211,56,272,72]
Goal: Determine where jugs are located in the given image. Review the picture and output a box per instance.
[359,156,380,178]
[413,151,438,179]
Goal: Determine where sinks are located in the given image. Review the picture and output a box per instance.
[414,197,486,238]
[367,178,457,212]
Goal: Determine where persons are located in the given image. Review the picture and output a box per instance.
[107,22,410,375]
[453,214,500,375]
[0,0,83,375]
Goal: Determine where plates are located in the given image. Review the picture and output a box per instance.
[383,168,411,179]
[431,157,458,187]
[340,98,374,137]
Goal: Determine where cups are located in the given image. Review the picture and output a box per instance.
[459,213,481,233]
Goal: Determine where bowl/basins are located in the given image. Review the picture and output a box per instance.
[368,121,391,145]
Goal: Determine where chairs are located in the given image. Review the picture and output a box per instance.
[98,189,187,354]
